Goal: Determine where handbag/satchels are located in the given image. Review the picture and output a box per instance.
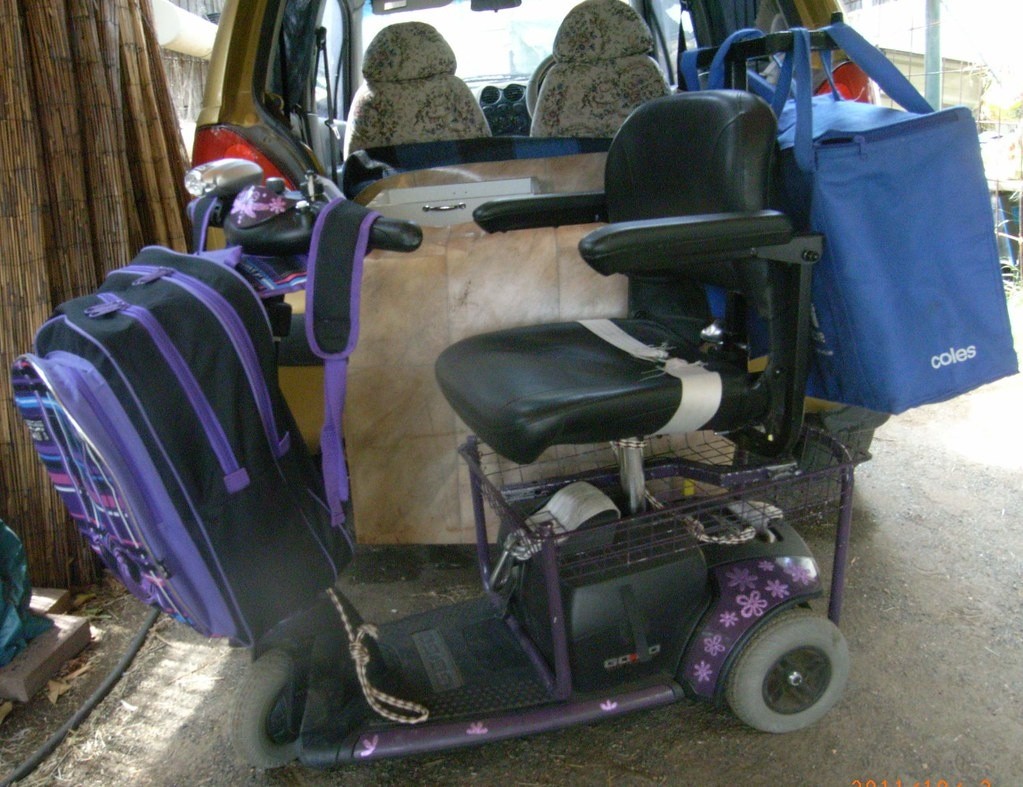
[684,26,1019,416]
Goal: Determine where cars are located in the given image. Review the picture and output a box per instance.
[185,0,861,458]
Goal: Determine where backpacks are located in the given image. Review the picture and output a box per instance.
[9,194,381,659]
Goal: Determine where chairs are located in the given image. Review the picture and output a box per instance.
[343,22,492,163]
[527,3,675,140]
[428,84,780,470]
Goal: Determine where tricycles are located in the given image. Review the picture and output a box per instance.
[186,10,852,769]
